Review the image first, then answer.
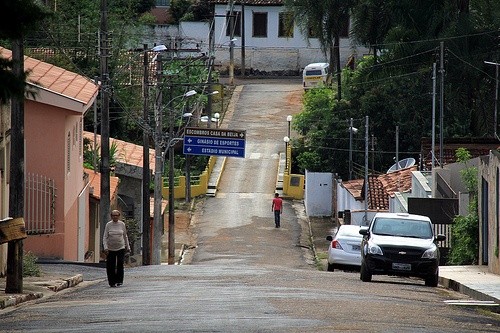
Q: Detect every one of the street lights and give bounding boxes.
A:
[99,44,168,256]
[141,89,221,267]
[283,136,290,169]
[286,114,293,140]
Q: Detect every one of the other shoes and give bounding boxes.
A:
[275,225,280,228]
[117,283,123,287]
[110,285,116,287]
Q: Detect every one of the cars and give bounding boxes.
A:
[325,224,369,272]
[359,212,447,288]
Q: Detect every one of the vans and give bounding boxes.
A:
[302,63,330,91]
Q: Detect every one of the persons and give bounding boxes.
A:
[103,210,131,287]
[272,193,283,228]
